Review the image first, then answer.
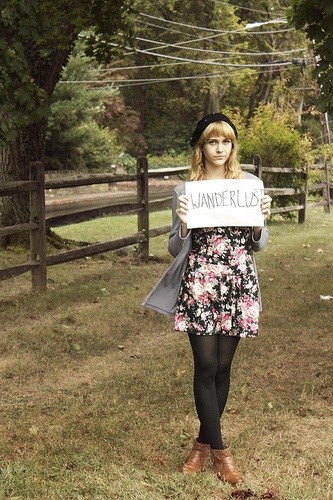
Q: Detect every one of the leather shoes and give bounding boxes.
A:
[181,437,244,486]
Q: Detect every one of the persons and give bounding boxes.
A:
[142,113,272,486]
